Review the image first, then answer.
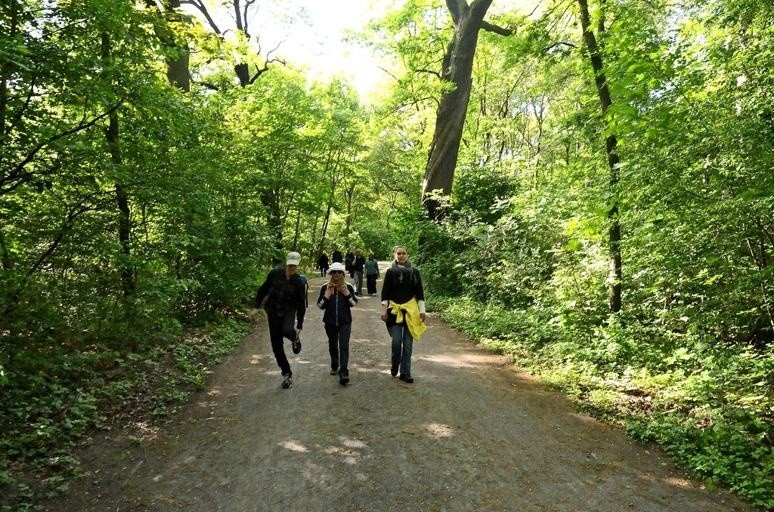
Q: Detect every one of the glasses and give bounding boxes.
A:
[331,271,343,274]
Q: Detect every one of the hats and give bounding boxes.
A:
[286,252,301,266]
[326,262,351,278]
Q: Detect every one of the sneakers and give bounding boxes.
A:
[399,374,413,383]
[292,335,301,354]
[391,365,398,377]
[330,368,338,375]
[368,293,376,297]
[340,376,349,384]
[281,372,292,388]
[355,292,362,297]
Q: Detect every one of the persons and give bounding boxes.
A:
[332,248,342,264]
[365,252,380,297]
[350,249,366,298]
[380,245,426,384]
[316,262,359,387]
[249,251,307,390]
[344,249,353,273]
[348,252,355,279]
[318,250,329,277]
